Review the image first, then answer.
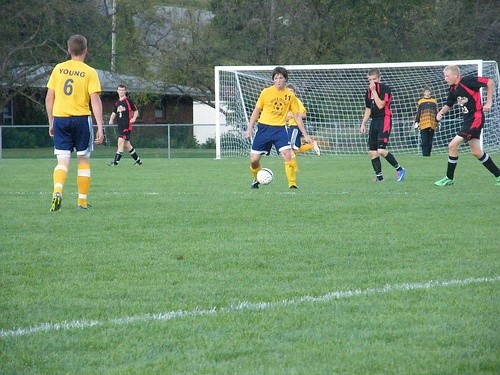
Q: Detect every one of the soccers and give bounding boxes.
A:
[256,167,275,186]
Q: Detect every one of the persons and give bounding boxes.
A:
[415,86,441,156]
[361,70,406,182]
[108,85,143,166]
[46,35,104,212]
[245,67,321,188]
[434,65,500,186]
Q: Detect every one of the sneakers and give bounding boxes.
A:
[49,191,62,212]
[76,203,92,211]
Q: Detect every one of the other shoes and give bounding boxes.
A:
[134,161,142,165]
[312,141,320,156]
[105,161,118,167]
[496,176,500,186]
[251,177,260,189]
[289,185,298,188]
[434,176,454,187]
[372,175,384,182]
[396,169,406,181]
